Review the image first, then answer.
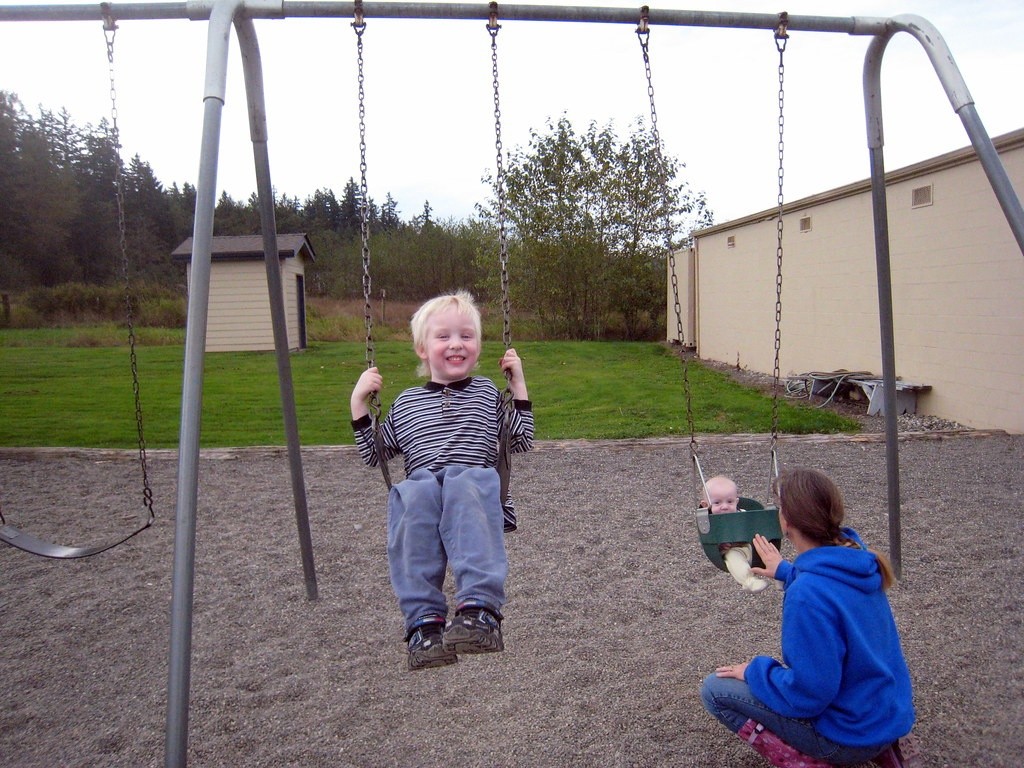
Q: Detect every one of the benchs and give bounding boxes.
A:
[805,374,932,417]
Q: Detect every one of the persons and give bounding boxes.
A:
[698,464,916,768]
[348,288,534,670]
[700,476,789,593]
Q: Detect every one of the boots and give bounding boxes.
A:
[872,731,921,768]
[739,719,831,768]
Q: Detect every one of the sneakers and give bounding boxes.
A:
[403,619,457,671]
[441,605,505,653]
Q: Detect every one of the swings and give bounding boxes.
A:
[0,23,158,560]
[639,32,784,574]
[355,26,522,534]
